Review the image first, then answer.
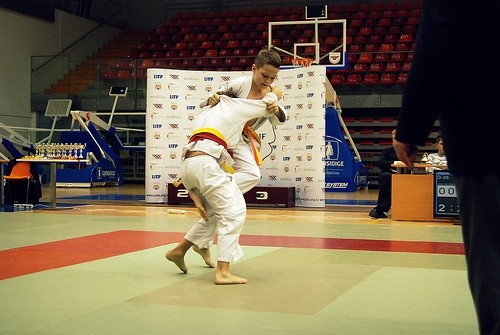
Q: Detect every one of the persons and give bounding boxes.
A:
[392,0,500,335]
[188,50,287,268]
[425,135,448,174]
[368,126,417,218]
[165,86,283,285]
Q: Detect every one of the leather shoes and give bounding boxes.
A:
[369,208,388,218]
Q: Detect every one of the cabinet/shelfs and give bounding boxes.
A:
[391,163,434,221]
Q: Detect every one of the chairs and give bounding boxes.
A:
[3,162,31,204]
[100,0,440,175]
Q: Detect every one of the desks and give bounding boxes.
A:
[16,159,89,210]
